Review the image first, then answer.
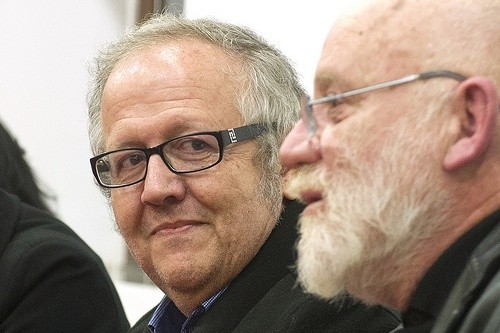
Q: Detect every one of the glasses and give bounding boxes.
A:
[305,70,468,140]
[90,123,277,188]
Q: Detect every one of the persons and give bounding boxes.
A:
[0,123,132,333]
[276,0,500,333]
[85,13,400,333]
